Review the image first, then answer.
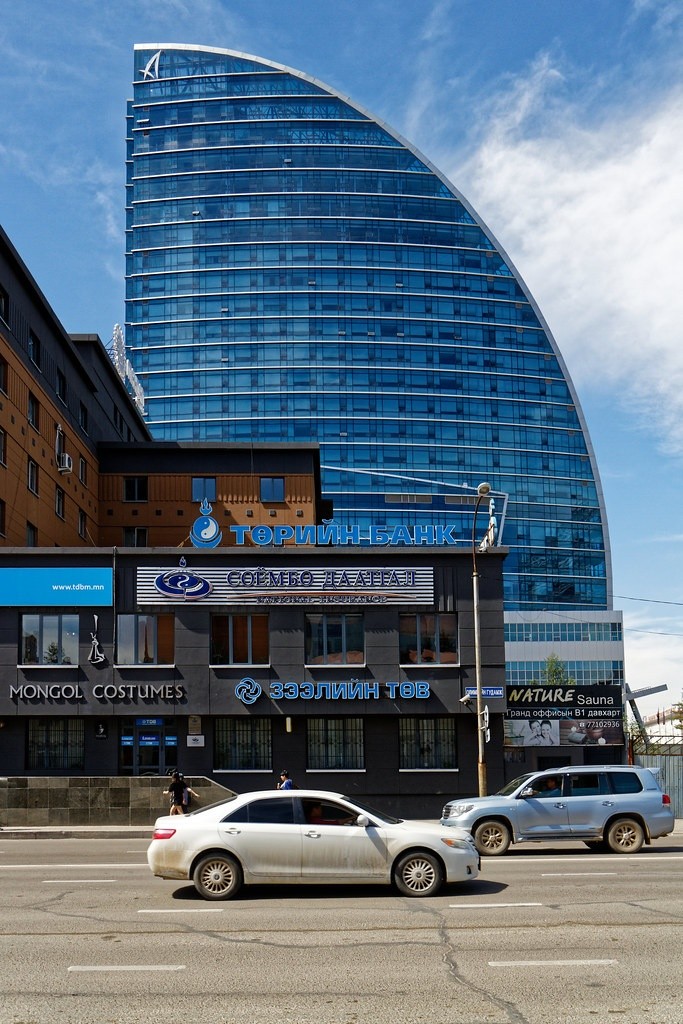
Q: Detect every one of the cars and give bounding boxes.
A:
[147,789,481,898]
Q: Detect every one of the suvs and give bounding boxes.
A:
[439,765,674,853]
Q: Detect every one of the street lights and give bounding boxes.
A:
[471,482,491,798]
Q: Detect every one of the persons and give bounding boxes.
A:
[521,720,543,744]
[163,771,200,815]
[177,772,189,814]
[526,720,558,745]
[302,799,350,827]
[276,769,293,789]
[530,775,562,799]
